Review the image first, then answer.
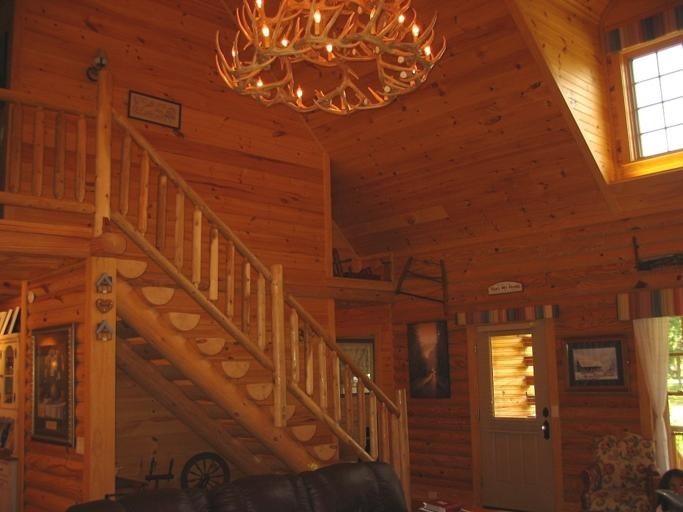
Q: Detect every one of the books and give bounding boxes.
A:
[422,498,463,512]
[0,306,20,336]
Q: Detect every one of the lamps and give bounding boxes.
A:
[214,0,447,115]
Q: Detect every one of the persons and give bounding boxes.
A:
[654,469,683,512]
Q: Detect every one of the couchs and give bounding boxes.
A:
[66,461,407,512]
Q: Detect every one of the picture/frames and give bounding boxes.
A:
[337,339,374,398]
[562,335,629,392]
[407,320,450,398]
[30,321,75,449]
[128,90,181,129]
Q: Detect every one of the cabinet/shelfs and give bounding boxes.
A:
[0,333,20,512]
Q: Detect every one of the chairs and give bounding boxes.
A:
[578,431,660,512]
